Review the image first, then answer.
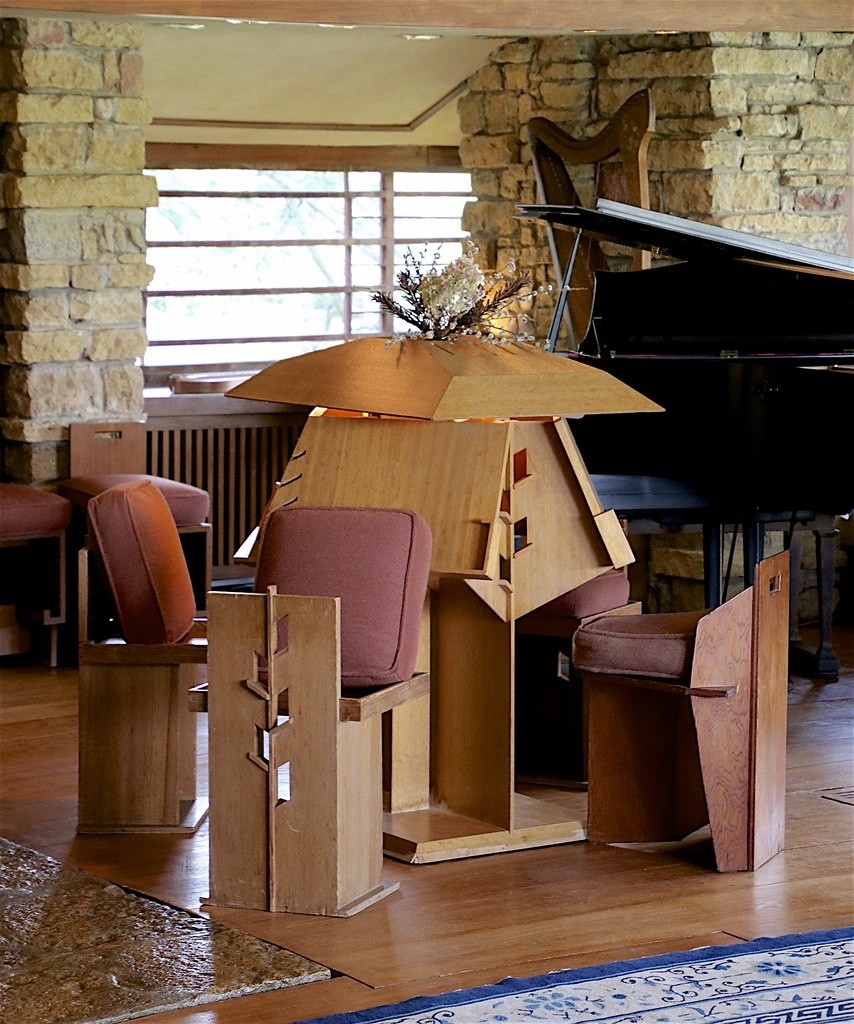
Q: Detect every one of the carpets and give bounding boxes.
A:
[295,926,854,1023]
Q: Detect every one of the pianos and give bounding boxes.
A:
[527,200,853,685]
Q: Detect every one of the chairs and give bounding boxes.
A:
[0,418,790,917]
